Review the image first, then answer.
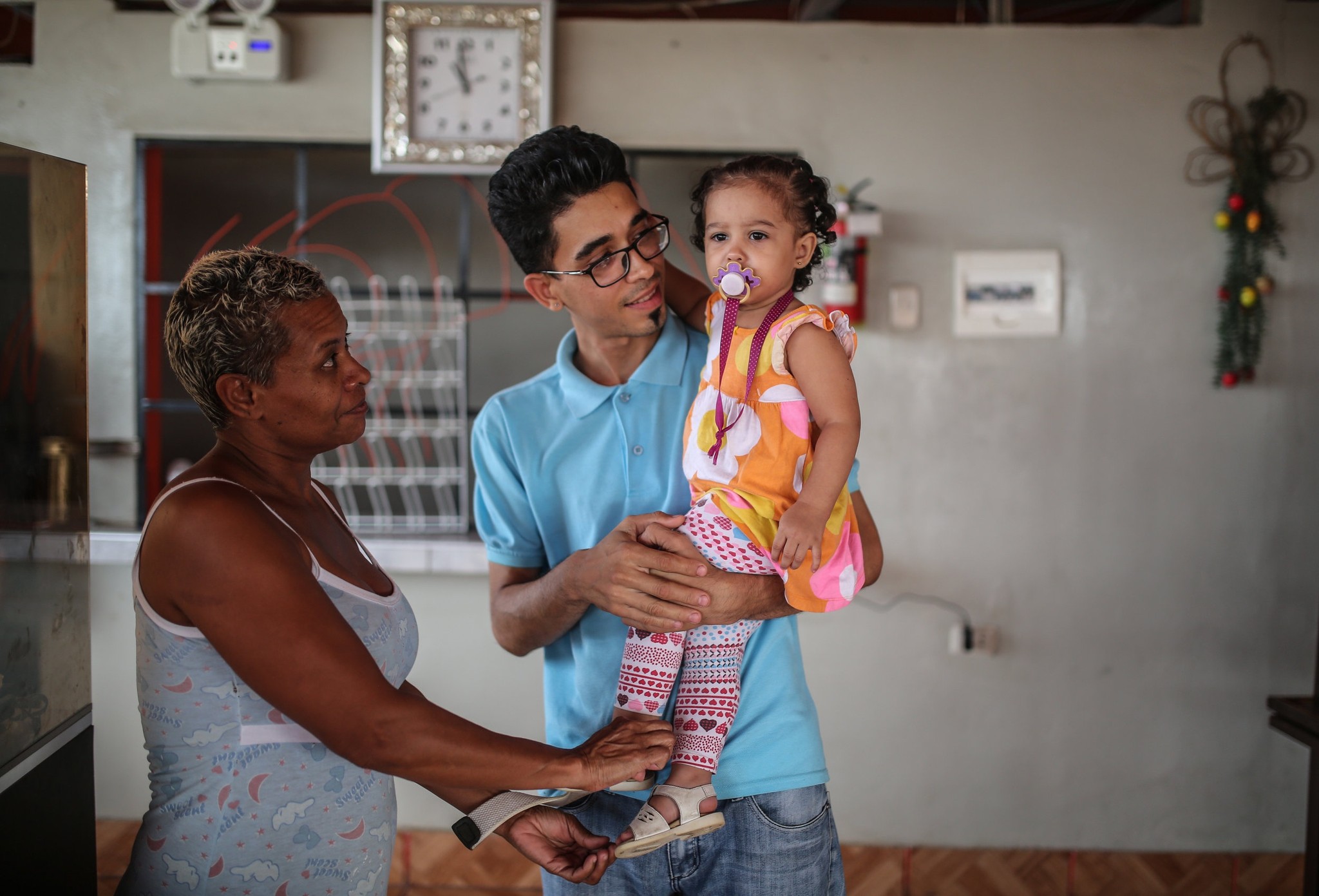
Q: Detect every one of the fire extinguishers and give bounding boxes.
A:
[817,176,877,322]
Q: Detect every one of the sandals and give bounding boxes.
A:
[614,784,725,858]
[554,772,655,792]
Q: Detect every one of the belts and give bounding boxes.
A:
[451,792,570,850]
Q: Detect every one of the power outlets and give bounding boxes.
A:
[950,626,1001,657]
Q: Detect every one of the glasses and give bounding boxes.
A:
[538,214,670,288]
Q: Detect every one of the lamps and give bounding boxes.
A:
[164,0,292,83]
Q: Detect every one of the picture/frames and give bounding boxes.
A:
[370,1,554,176]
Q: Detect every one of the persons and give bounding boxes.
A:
[558,156,861,858]
[471,123,883,896]
[117,247,675,896]
